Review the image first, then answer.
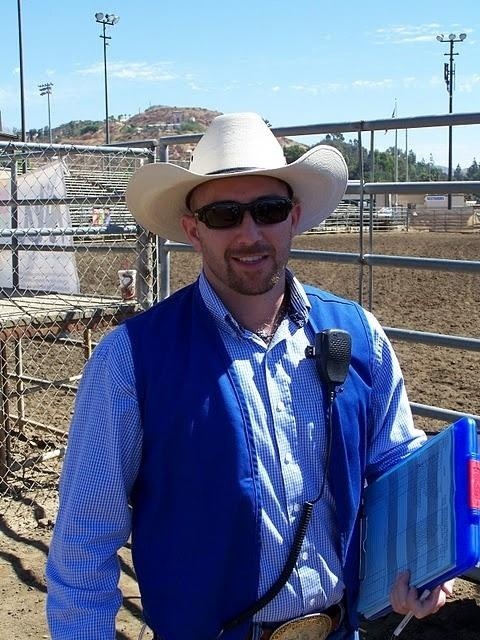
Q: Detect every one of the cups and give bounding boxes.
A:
[118,270,138,301]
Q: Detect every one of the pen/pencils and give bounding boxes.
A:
[390,589,432,639]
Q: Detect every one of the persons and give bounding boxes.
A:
[43,112,455,639]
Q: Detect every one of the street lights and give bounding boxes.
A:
[40,82,55,141]
[93,10,119,144]
[436,31,466,207]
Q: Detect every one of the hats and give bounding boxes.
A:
[125,112,349,246]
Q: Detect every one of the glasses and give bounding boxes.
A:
[197,194,292,229]
